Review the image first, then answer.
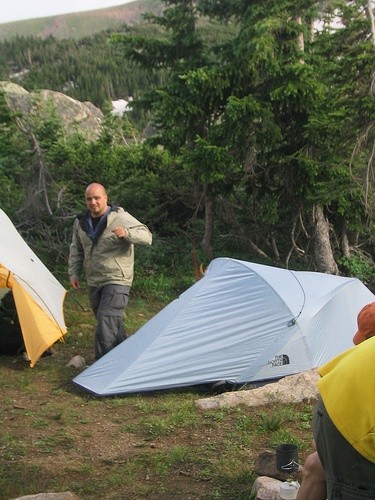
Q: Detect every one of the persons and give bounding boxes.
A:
[68,182,153,359]
[294,302,375,500]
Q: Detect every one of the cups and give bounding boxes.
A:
[276,444,298,471]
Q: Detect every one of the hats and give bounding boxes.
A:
[353,301,375,345]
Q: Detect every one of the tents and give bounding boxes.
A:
[0,208,87,368]
[72,257,375,395]
